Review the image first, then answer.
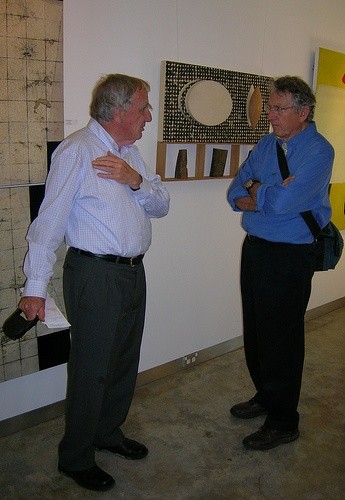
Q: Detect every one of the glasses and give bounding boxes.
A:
[264,105,295,113]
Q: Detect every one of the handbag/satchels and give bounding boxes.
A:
[312,221,343,271]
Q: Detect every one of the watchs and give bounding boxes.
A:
[245,179,259,192]
[132,176,143,191]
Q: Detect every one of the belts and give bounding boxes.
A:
[72,246,143,267]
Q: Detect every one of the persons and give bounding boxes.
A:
[226,75,334,449]
[20,74,170,492]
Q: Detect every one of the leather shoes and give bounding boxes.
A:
[229,393,266,418]
[60,465,115,490]
[95,435,148,460]
[242,423,300,450]
